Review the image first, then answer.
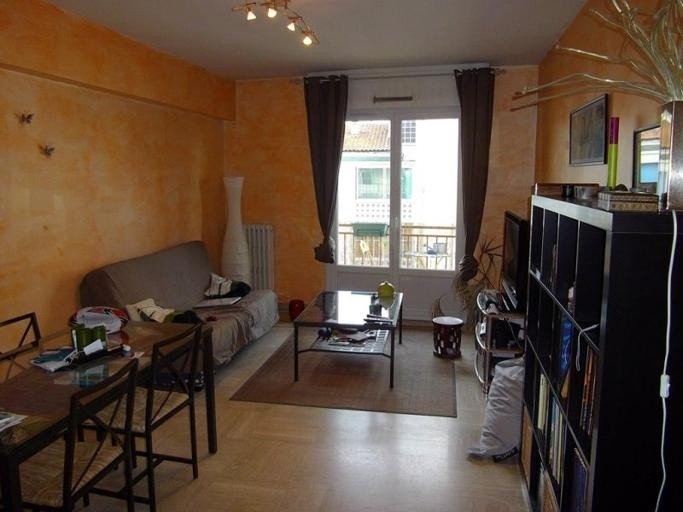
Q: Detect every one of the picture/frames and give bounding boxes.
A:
[568,92,608,168]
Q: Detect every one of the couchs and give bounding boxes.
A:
[79,240,280,393]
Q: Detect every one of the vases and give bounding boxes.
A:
[220,175,253,289]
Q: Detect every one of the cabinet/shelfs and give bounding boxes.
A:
[474,290,525,393]
[517,194,683,512]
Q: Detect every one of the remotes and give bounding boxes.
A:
[364,314,393,323]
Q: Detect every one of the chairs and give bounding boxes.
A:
[1,312,77,442]
[16,356,140,511]
[74,319,203,511]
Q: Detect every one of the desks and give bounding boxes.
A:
[2,318,214,505]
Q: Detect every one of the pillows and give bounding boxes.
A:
[125,298,174,323]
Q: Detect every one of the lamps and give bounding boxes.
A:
[231,0,320,47]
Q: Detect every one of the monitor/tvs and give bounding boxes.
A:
[499,211,528,312]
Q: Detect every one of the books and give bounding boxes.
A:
[538,345,598,485]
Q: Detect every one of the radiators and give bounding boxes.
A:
[242,223,276,291]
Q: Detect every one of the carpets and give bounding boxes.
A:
[229,324,458,419]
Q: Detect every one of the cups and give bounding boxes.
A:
[563,184,593,201]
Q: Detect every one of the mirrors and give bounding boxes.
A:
[631,122,661,193]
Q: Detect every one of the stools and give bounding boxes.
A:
[432,316,464,360]
